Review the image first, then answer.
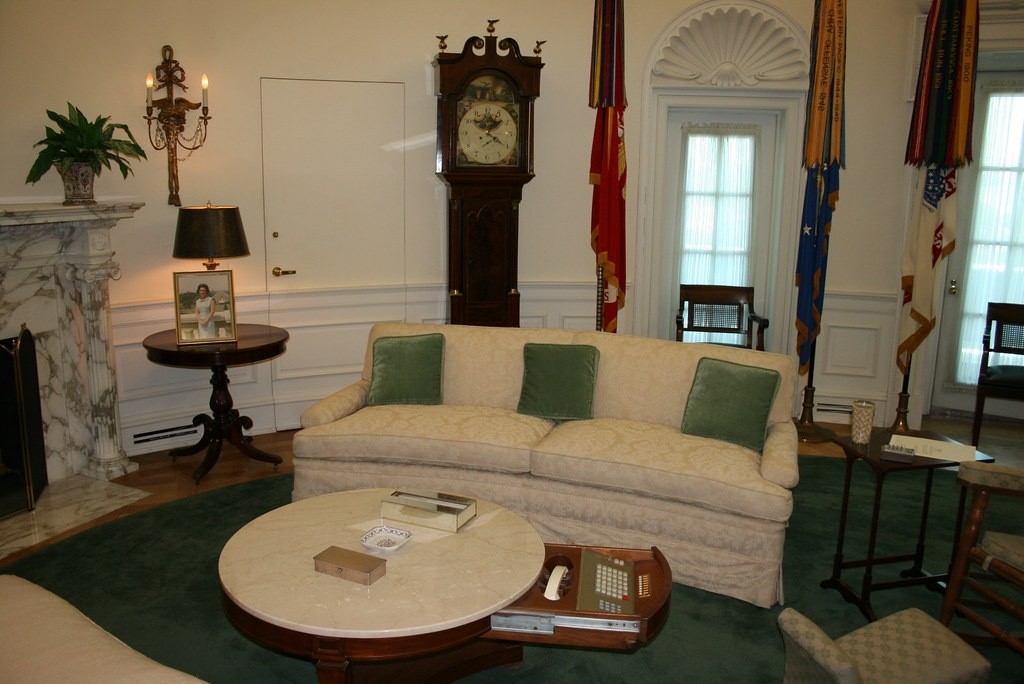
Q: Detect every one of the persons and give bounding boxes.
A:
[195,283,217,340]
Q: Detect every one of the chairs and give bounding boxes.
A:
[777,604,992,684]
[939,461,1024,661]
[972,302,1024,449]
[675,284,770,351]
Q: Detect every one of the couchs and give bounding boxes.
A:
[0,576,211,684]
[292,320,799,609]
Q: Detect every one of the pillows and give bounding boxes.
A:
[517,344,600,422]
[681,357,782,455]
[365,331,445,408]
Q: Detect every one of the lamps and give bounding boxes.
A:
[139,45,212,205]
[172,200,251,271]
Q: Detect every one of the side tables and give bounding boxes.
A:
[820,429,995,627]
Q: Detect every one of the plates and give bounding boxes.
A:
[360,525,412,553]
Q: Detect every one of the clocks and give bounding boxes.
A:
[429,19,547,328]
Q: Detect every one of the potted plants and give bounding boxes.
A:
[26,101,148,208]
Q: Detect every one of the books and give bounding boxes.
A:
[888,434,976,463]
[380,487,478,534]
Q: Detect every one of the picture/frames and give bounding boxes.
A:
[173,270,239,346]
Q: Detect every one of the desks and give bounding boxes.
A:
[143,323,289,486]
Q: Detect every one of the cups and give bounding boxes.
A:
[851,399,875,444]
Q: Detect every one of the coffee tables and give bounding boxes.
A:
[217,488,674,684]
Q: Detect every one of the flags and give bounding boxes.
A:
[793,0,847,377]
[894,0,981,373]
[587,0,629,336]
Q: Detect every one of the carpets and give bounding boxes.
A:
[0,452,1024,684]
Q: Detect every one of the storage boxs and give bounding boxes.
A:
[313,546,388,586]
